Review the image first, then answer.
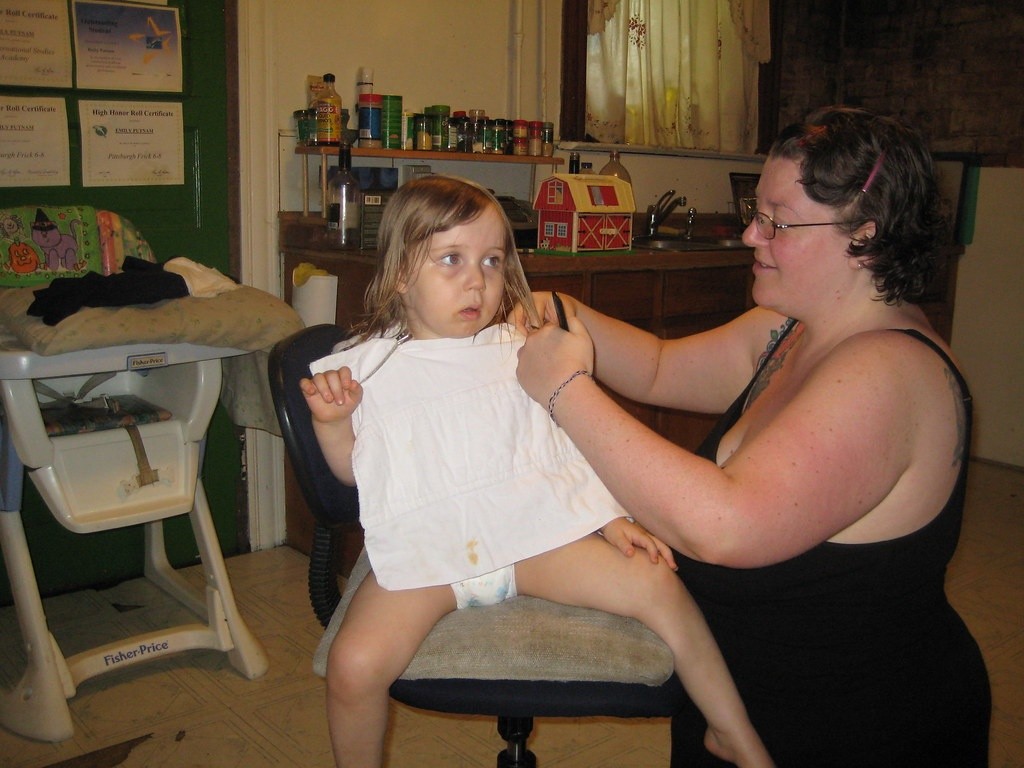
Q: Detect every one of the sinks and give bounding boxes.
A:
[634,239,726,251]
[693,238,748,248]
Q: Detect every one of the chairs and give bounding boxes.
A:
[266,321,692,768]
[1,205,267,746]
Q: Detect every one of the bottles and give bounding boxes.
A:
[293,67,554,157]
[568,151,580,176]
[579,162,596,175]
[599,152,632,189]
[325,141,361,249]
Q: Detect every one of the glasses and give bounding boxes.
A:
[738,195,870,240]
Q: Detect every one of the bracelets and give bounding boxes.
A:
[547,370,591,429]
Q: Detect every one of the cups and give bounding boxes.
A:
[739,198,757,226]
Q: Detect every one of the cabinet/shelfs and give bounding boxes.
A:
[279,146,758,453]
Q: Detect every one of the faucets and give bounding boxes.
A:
[645,189,687,237]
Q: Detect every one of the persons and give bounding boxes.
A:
[298,171,780,768]
[511,104,994,768]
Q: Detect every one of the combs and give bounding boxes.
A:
[551,290,570,332]
